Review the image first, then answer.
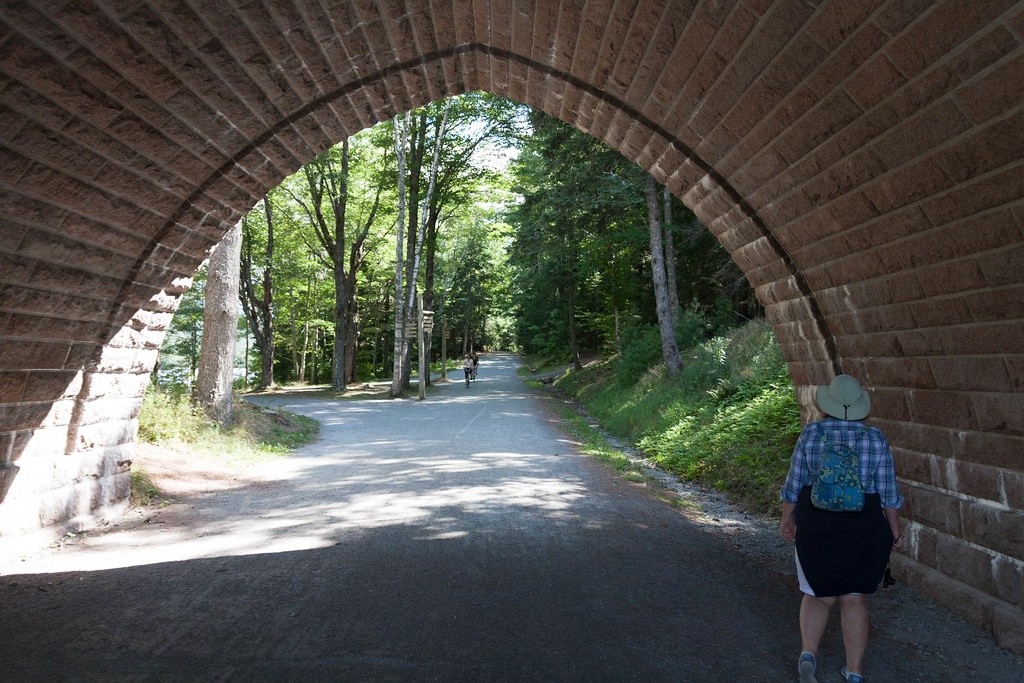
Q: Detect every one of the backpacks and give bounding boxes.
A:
[807,421,876,510]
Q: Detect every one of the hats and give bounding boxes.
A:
[816,373,871,420]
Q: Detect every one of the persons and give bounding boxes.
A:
[463,352,478,385]
[781,374,905,683]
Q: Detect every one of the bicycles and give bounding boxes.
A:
[463,367,471,388]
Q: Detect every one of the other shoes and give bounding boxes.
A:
[799,652,817,683]
[841,666,862,683]
